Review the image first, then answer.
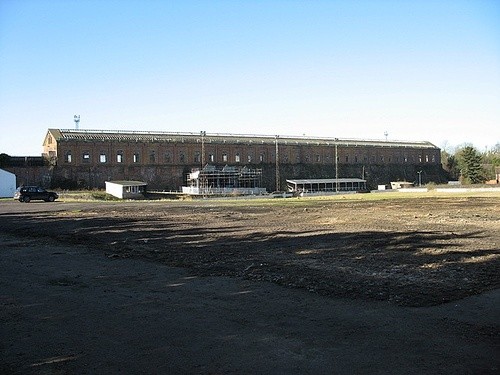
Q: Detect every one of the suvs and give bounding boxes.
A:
[14,186,59,203]
